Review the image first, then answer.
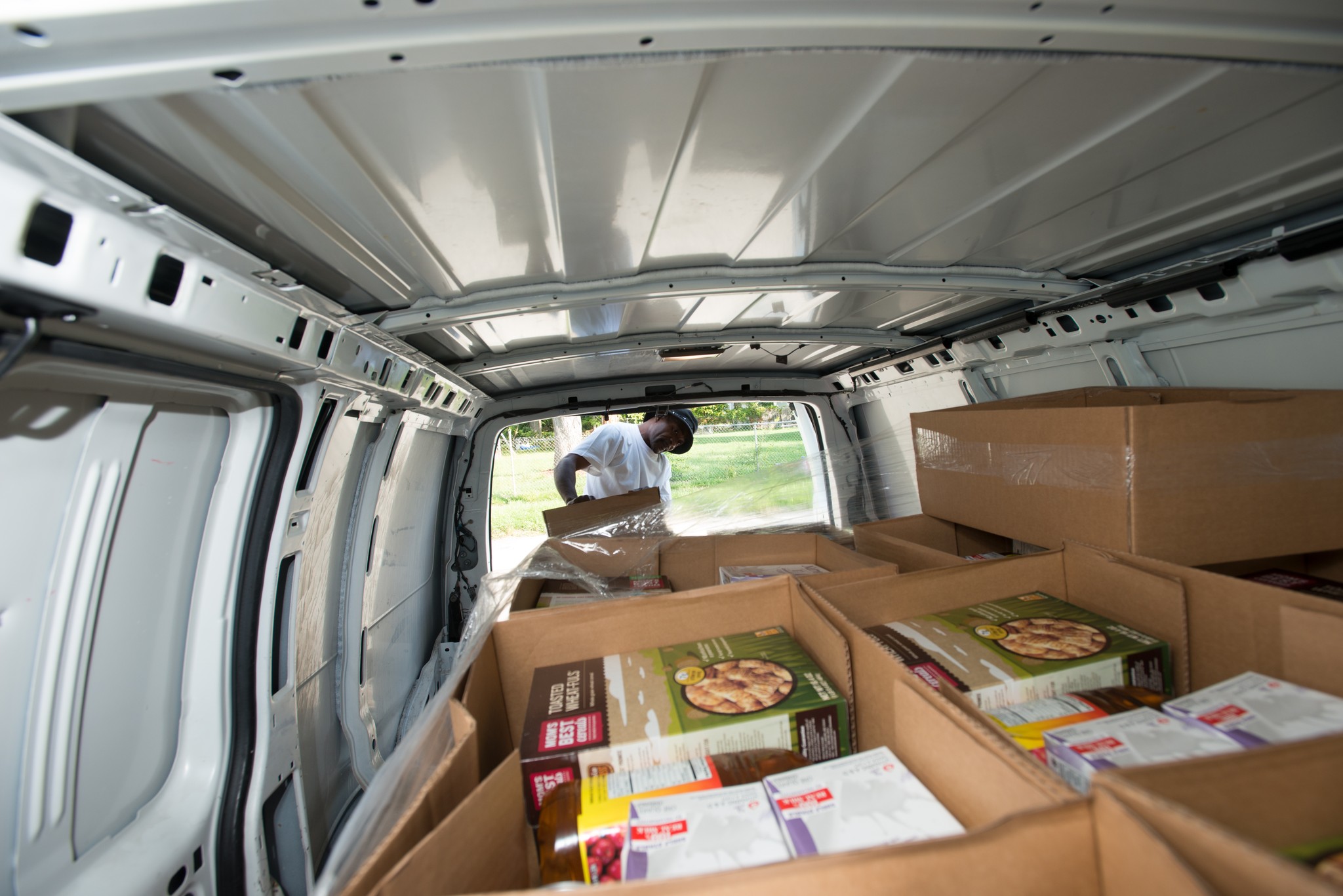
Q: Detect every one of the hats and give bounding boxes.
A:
[642,408,698,455]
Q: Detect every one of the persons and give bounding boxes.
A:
[553,407,697,509]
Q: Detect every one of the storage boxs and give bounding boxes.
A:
[340,385,1341,894]
